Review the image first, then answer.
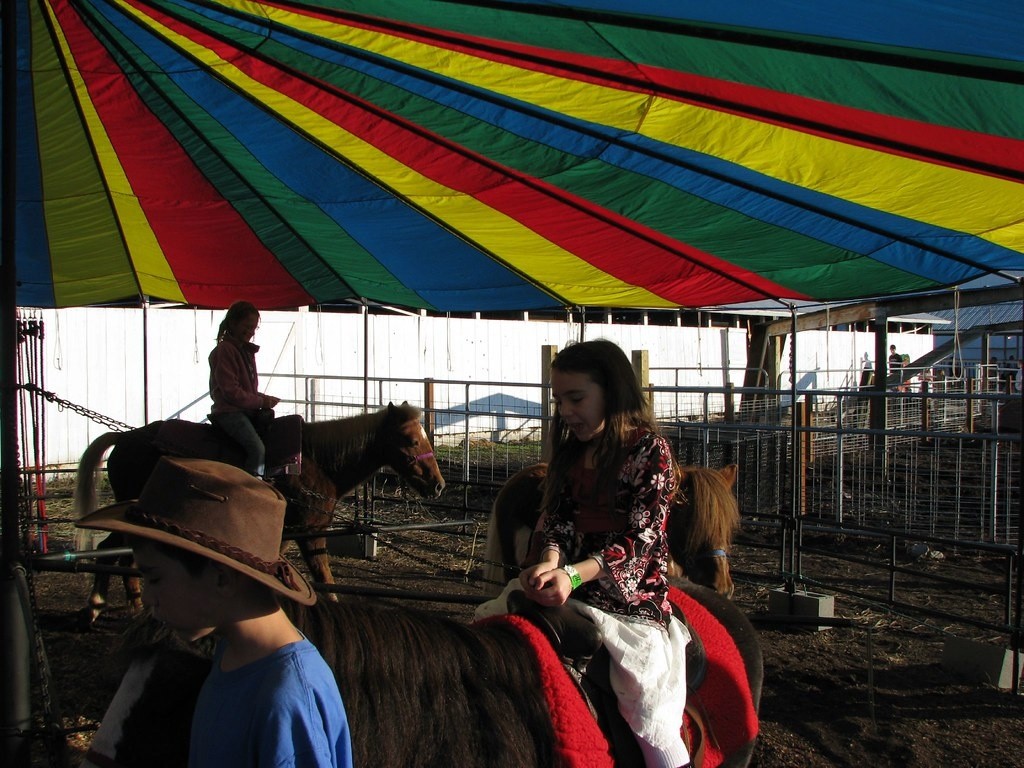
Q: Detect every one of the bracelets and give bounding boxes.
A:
[563,565,583,590]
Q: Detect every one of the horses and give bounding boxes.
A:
[485,463,739,599]
[73,402,446,629]
[77,587,763,768]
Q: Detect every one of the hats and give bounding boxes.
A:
[74,455,317,605]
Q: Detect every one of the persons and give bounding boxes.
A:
[74,453,353,768]
[471,340,693,767]
[948,354,1023,394]
[889,345,903,373]
[208,302,279,477]
